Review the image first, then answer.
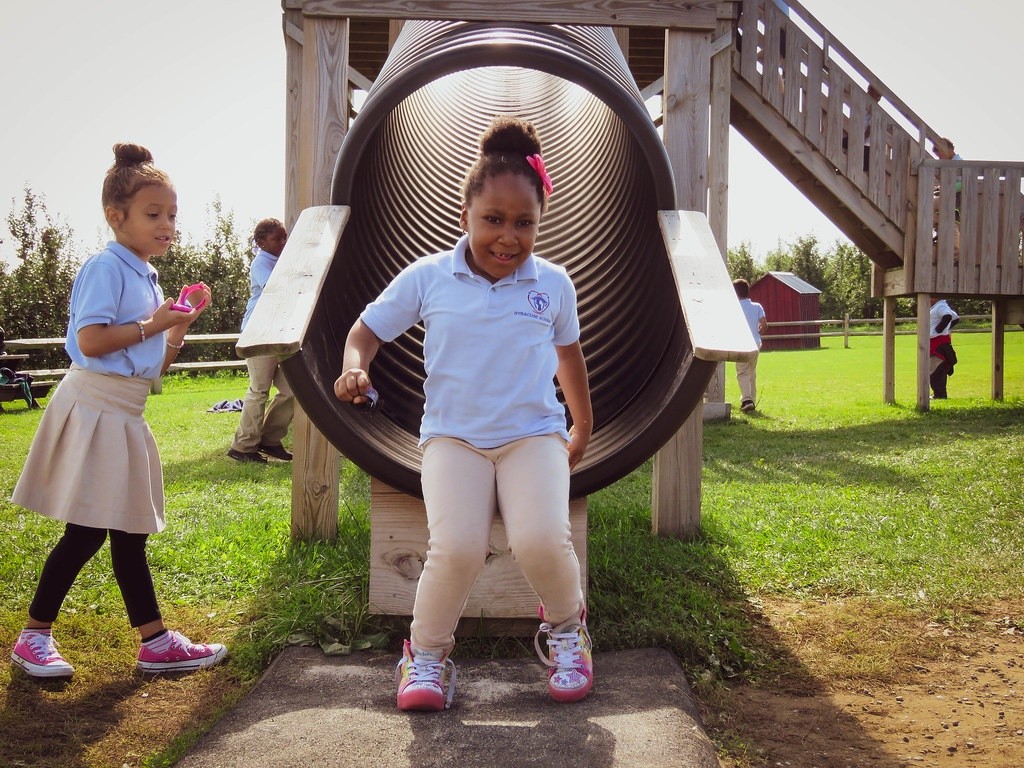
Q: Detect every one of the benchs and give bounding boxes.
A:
[0,381,58,414]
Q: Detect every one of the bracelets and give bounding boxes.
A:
[137,321,145,343]
[167,340,185,349]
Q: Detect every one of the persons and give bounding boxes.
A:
[226,220,295,463]
[732,278,769,412]
[334,115,593,709]
[928,294,959,400]
[10,140,227,678]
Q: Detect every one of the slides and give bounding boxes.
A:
[280,18,719,501]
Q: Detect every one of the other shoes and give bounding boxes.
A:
[740,400,755,411]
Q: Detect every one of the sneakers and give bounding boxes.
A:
[136,632,228,673]
[534,601,593,701]
[257,444,293,460]
[395,639,456,710]
[10,633,76,677]
[226,448,267,464]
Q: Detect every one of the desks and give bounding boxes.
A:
[0,354,29,380]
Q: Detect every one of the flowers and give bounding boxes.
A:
[526,154,553,194]
